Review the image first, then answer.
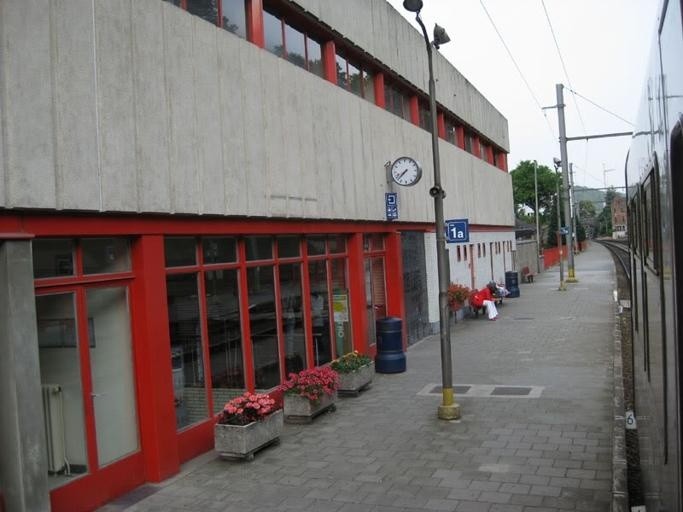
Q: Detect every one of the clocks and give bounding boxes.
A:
[389,155,423,187]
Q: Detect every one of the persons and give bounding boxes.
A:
[470,289,499,321]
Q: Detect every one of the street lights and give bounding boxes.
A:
[550,155,570,290]
[399,0,466,424]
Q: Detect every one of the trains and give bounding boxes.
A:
[620,0,682,512]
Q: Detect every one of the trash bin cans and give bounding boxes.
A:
[505,271,520,297]
[374,316,406,373]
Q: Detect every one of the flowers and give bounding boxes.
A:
[267,365,340,407]
[214,388,278,427]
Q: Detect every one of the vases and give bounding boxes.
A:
[273,381,338,425]
[204,406,285,464]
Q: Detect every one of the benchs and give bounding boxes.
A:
[466,282,506,317]
[521,265,537,282]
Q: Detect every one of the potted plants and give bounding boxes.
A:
[328,348,377,403]
